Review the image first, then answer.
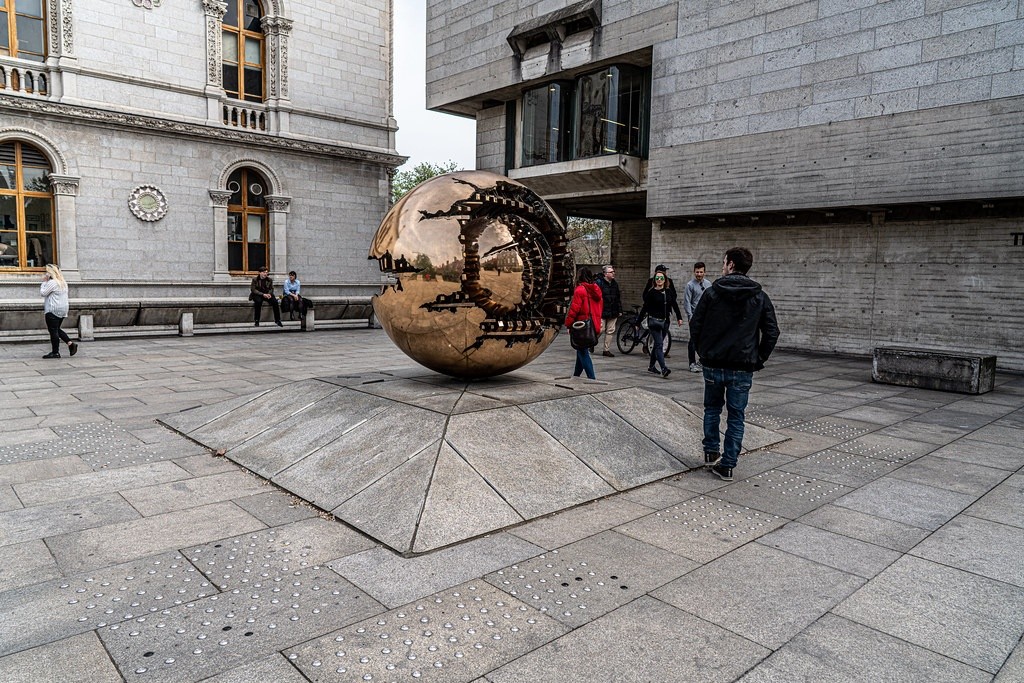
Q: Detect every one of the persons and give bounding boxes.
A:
[589,264,623,357]
[426,273,430,280]
[634,270,684,378]
[283,271,304,320]
[248,267,283,327]
[642,264,677,358]
[684,262,713,373]
[498,268,500,276]
[564,266,603,380]
[0,243,18,267]
[40,263,78,359]
[689,246,780,480]
[27,235,50,267]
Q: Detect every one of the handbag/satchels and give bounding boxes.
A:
[648,317,666,330]
[570,318,598,351]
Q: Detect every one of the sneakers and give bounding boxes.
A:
[712,465,733,480]
[704,452,720,464]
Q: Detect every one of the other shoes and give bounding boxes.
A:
[665,354,670,358]
[643,346,648,354]
[589,346,594,353]
[43,352,60,358]
[256,321,259,326]
[602,351,614,357]
[648,365,661,374]
[275,321,283,327]
[689,363,699,373]
[662,366,671,377]
[696,362,703,372]
[69,343,77,356]
[290,315,295,320]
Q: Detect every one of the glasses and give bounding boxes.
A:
[606,270,615,273]
[699,282,705,291]
[655,276,664,281]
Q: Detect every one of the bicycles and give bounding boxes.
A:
[617,304,672,358]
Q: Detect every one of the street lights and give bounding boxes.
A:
[412,252,419,266]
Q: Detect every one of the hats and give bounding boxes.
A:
[655,265,669,271]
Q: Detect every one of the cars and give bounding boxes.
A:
[484,263,494,271]
